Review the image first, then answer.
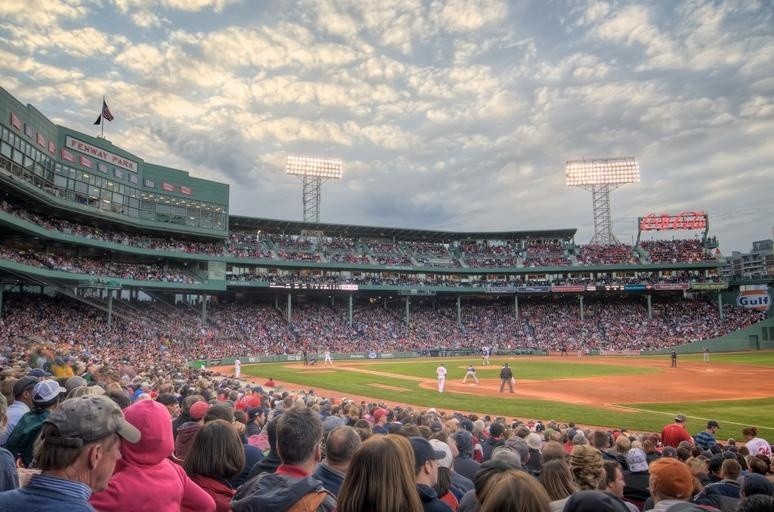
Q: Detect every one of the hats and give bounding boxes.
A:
[245,407,262,424]
[570,444,603,465]
[66,376,87,390]
[728,437,735,443]
[454,429,473,449]
[182,394,204,407]
[568,429,576,439]
[28,368,51,377]
[707,420,720,429]
[571,430,585,443]
[428,438,452,468]
[408,436,445,466]
[189,401,212,418]
[157,393,175,405]
[66,386,95,398]
[678,440,690,449]
[648,457,692,497]
[218,389,223,394]
[543,428,555,439]
[101,370,112,376]
[649,433,660,444]
[662,446,676,457]
[626,447,648,471]
[709,455,721,470]
[373,407,388,416]
[141,381,150,387]
[31,379,65,402]
[13,375,38,395]
[526,432,542,455]
[41,394,141,448]
[102,381,129,398]
[322,415,345,433]
[562,488,629,512]
[432,422,441,431]
[723,458,739,473]
[254,385,266,394]
[674,414,686,421]
[492,446,521,465]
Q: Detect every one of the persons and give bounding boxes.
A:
[480,469,549,512]
[459,446,520,512]
[0,379,38,447]
[0,392,20,491]
[474,460,509,504]
[90,399,216,512]
[541,461,574,502]
[4,378,66,467]
[234,357,241,377]
[452,430,481,482]
[593,414,738,458]
[463,364,479,384]
[499,362,515,393]
[694,459,742,512]
[406,437,453,512]
[549,444,605,512]
[175,401,212,460]
[232,407,338,512]
[246,406,266,437]
[505,436,540,476]
[738,453,774,512]
[182,419,246,512]
[601,460,626,498]
[457,412,593,448]
[623,448,650,502]
[1,184,768,364]
[322,393,457,436]
[428,438,459,512]
[336,434,424,512]
[311,426,361,497]
[644,458,693,512]
[0,352,235,377]
[644,458,722,512]
[741,427,772,458]
[68,377,322,411]
[436,363,447,392]
[0,394,141,512]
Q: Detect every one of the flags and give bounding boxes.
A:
[104,100,114,122]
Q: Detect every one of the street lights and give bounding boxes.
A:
[286,155,343,222]
[566,161,640,244]
